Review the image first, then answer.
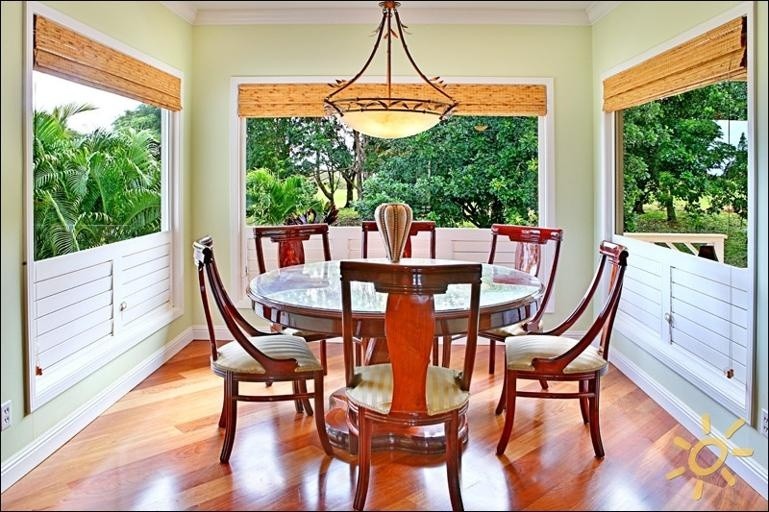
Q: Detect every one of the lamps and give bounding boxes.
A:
[324,1,458,139]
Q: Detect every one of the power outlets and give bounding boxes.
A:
[1,400,12,430]
[760,408,769,438]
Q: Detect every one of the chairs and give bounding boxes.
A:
[356,221,438,366]
[192,235,334,463]
[253,224,361,386]
[496,241,629,457]
[325,260,482,511]
[442,224,563,389]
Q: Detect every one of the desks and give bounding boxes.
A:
[246,258,546,454]
